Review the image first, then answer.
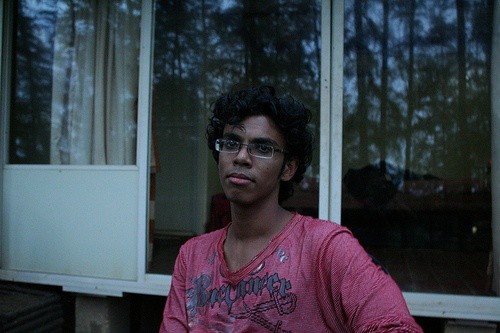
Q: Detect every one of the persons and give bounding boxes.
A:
[157,71,428,333]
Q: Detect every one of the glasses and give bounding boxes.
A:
[215,138,284,157]
